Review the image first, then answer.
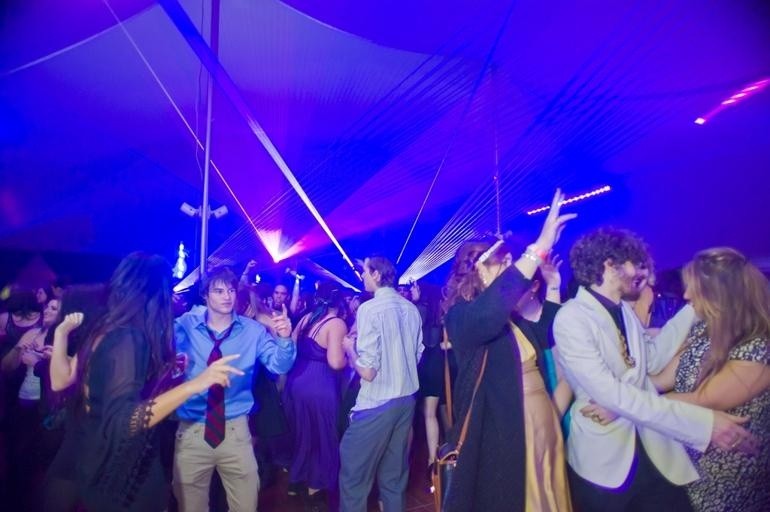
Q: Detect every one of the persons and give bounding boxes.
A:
[443,188,576,511]
[550,228,770,511]
[238,255,445,511]
[0,251,296,512]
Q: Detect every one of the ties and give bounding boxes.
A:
[202,326,234,449]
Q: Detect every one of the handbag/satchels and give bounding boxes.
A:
[429,442,470,508]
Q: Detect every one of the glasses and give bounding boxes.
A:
[211,288,239,296]
